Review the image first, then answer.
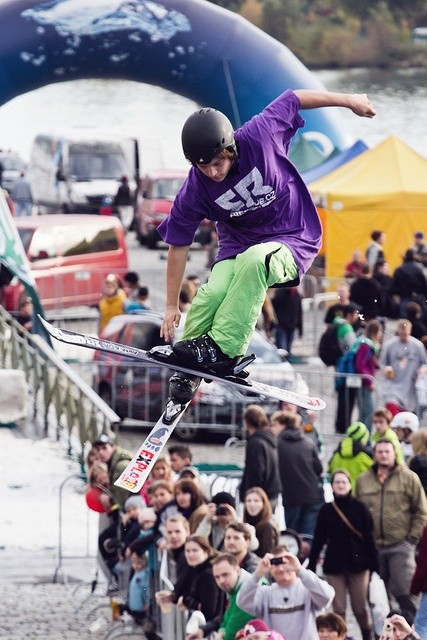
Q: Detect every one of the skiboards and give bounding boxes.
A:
[34,312,325,496]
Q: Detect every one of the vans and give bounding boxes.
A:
[0,148,28,194]
[0,213,131,320]
[91,309,315,441]
[136,172,213,249]
[28,132,137,218]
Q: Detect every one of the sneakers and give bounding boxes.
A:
[173,334,244,374]
[169,371,202,404]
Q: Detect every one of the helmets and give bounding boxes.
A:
[182,108,236,168]
[390,412,420,433]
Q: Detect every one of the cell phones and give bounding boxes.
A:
[270,557,283,565]
[216,507,227,516]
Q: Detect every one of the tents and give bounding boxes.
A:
[306,132,427,288]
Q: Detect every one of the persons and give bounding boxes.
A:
[177,535,228,620]
[356,438,427,622]
[195,502,238,552]
[307,470,378,640]
[168,278,193,348]
[178,466,200,487]
[407,429,427,493]
[97,275,125,339]
[222,522,262,574]
[185,553,271,640]
[154,515,191,603]
[151,89,376,376]
[88,461,111,494]
[129,551,155,639]
[316,612,353,640]
[115,176,134,234]
[123,271,141,300]
[137,507,158,578]
[405,302,427,345]
[329,422,374,500]
[412,231,426,266]
[11,172,34,217]
[245,618,286,640]
[394,249,427,319]
[351,265,384,367]
[377,610,420,640]
[277,397,322,455]
[124,286,151,313]
[175,479,211,534]
[372,257,403,365]
[270,284,303,357]
[13,296,35,394]
[87,451,99,468]
[187,274,201,294]
[243,487,280,557]
[370,406,406,467]
[168,445,200,483]
[236,545,335,640]
[146,484,162,513]
[375,318,427,429]
[393,412,418,470]
[138,457,176,508]
[365,230,386,267]
[235,629,251,640]
[117,495,149,581]
[334,303,362,435]
[322,283,363,332]
[343,250,372,284]
[154,480,177,561]
[237,405,280,524]
[358,319,384,433]
[93,434,133,595]
[206,218,220,268]
[270,411,326,554]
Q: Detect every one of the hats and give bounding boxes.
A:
[138,507,157,525]
[93,434,114,445]
[244,619,271,638]
[180,466,199,477]
[125,495,147,509]
[347,422,369,446]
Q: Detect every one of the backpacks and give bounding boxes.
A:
[335,343,371,395]
[319,324,344,364]
